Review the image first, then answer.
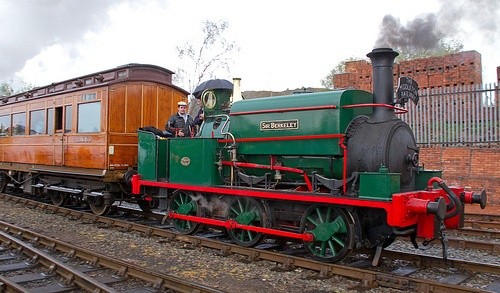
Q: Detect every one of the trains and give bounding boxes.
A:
[0,48,487,271]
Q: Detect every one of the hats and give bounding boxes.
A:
[177,101,187,105]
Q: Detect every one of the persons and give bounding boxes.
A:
[165,101,194,138]
[191,98,203,127]
[35,110,45,135]
